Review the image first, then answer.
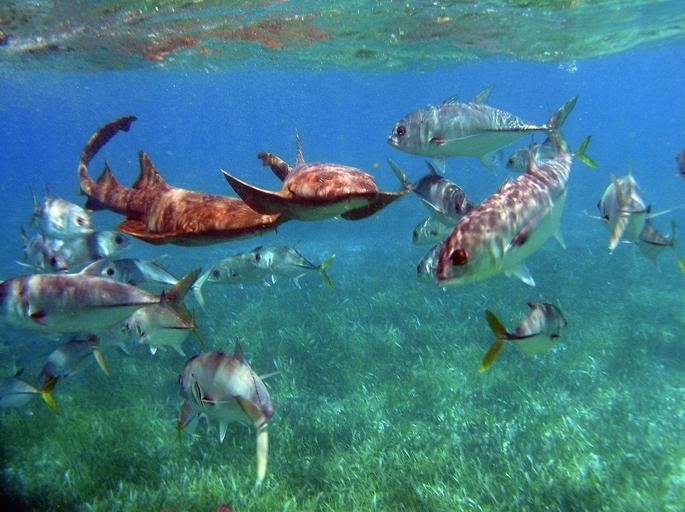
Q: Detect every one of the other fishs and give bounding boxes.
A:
[385,83,598,294]
[0,180,337,491]
